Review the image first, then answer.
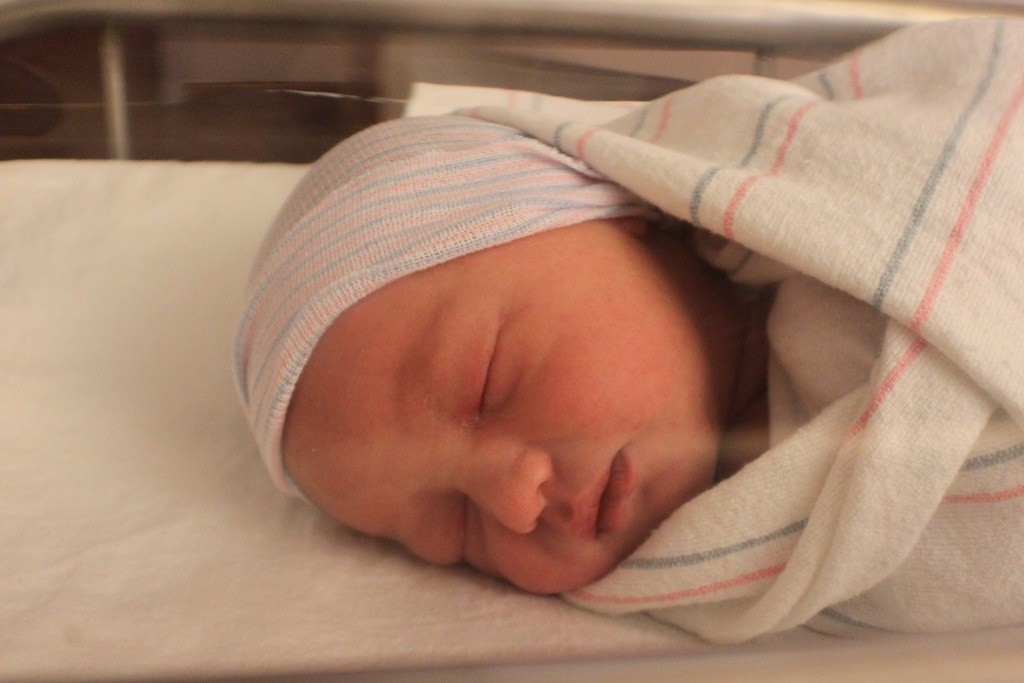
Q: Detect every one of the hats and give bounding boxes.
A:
[234,80,664,517]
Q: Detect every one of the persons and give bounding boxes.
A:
[230,15,1024,647]
